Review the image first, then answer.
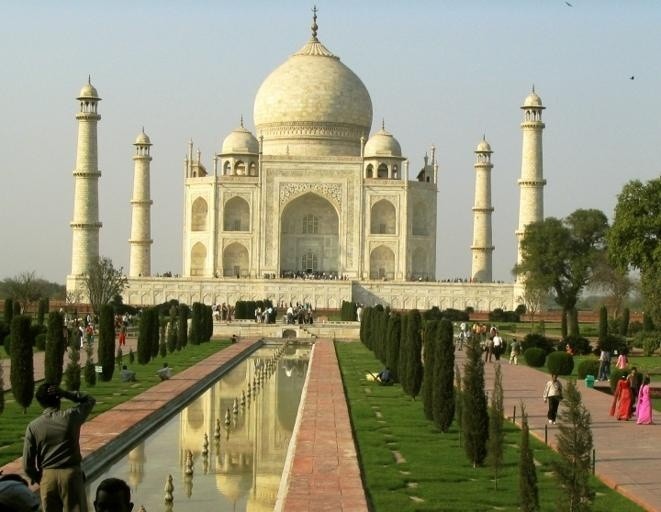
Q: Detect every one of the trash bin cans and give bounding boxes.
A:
[585,374,595,387]
[87,335,95,342]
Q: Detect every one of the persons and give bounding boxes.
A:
[608,372,633,422]
[615,350,628,370]
[138,270,516,284]
[373,366,396,386]
[119,364,136,384]
[155,362,170,380]
[542,372,563,426]
[92,478,134,511]
[624,367,639,414]
[563,342,575,356]
[633,374,654,425]
[211,300,314,324]
[229,333,238,344]
[603,347,611,382]
[452,319,520,366]
[356,303,366,322]
[19,382,95,512]
[0,472,40,511]
[62,304,149,352]
[593,348,607,382]
